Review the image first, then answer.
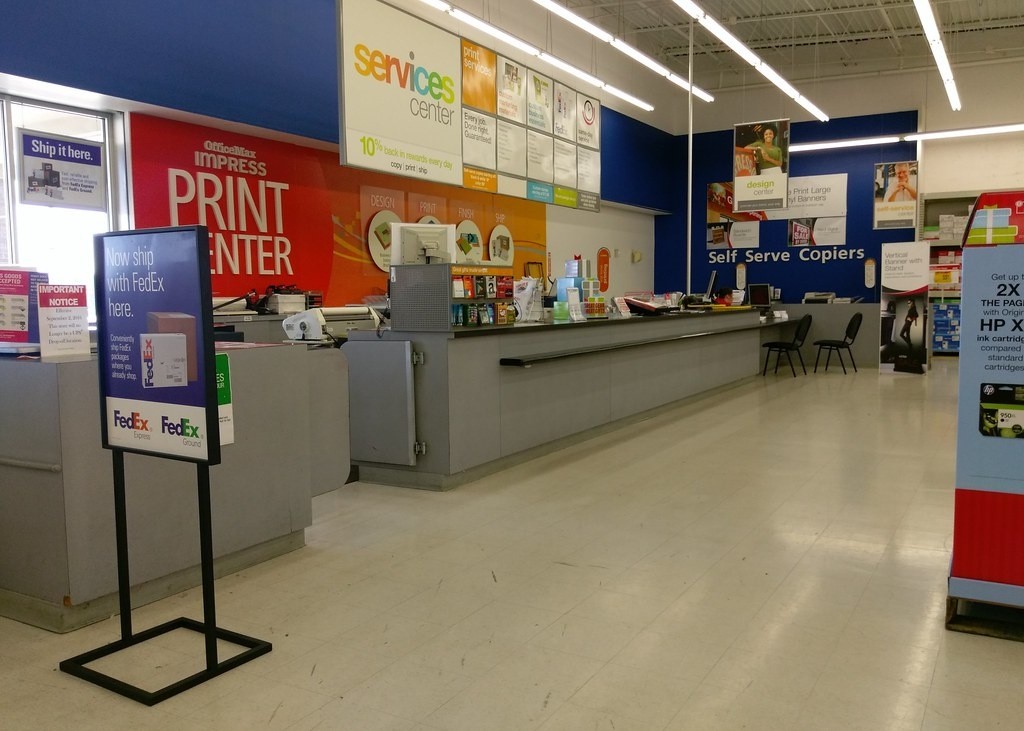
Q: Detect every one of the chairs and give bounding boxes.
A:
[761,313,812,377]
[812,312,863,375]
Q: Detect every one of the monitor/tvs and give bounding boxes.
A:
[392,222,456,265]
[706,271,719,299]
[748,283,771,308]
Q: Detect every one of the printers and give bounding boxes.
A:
[802,292,836,304]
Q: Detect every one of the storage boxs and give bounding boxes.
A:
[954,216,969,228]
[955,250,962,264]
[939,227,954,240]
[934,319,960,334]
[953,227,966,241]
[933,333,960,351]
[933,304,961,319]
[930,250,955,264]
[924,226,939,239]
[938,214,955,228]
[929,265,960,284]
[259,294,305,314]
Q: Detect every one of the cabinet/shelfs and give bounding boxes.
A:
[918,186,1024,353]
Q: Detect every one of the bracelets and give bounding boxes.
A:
[757,146,760,151]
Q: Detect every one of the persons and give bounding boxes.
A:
[899,299,919,350]
[745,126,785,175]
[877,163,917,202]
